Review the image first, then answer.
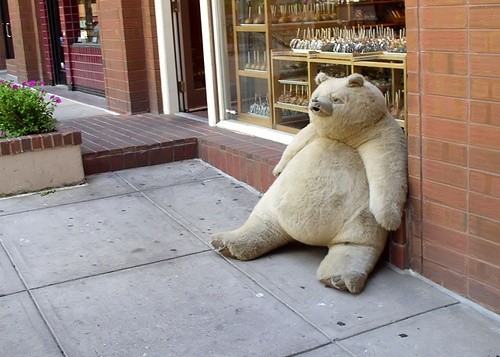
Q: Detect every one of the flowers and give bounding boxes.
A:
[0,77,62,137]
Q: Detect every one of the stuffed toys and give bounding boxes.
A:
[211,72,407,294]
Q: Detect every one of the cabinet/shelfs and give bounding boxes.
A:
[232,0,406,131]
[79,16,101,45]
[271,45,408,148]
